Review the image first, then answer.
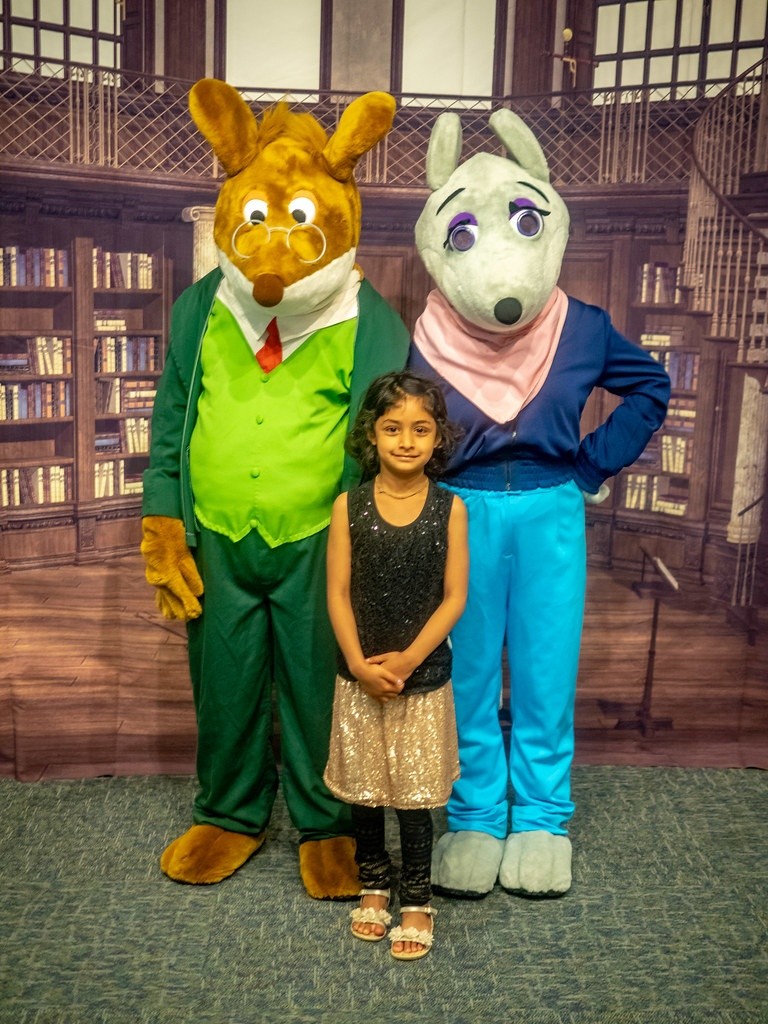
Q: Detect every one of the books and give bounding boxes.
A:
[1,466,72,506]
[638,259,685,304]
[663,398,697,430]
[625,473,688,517]
[640,323,684,347]
[94,459,143,498]
[94,308,126,332]
[95,377,157,412]
[649,350,700,392]
[637,435,694,476]
[95,417,148,454]
[0,336,71,375]
[93,241,154,289]
[93,336,158,372]
[0,244,70,287]
[0,380,72,420]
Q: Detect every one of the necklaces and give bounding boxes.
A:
[374,473,429,499]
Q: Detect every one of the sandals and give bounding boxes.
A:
[349,888,392,942]
[387,906,438,960]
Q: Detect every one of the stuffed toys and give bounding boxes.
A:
[403,107,672,900]
[139,76,411,901]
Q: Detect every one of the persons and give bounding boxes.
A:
[325,370,470,960]
